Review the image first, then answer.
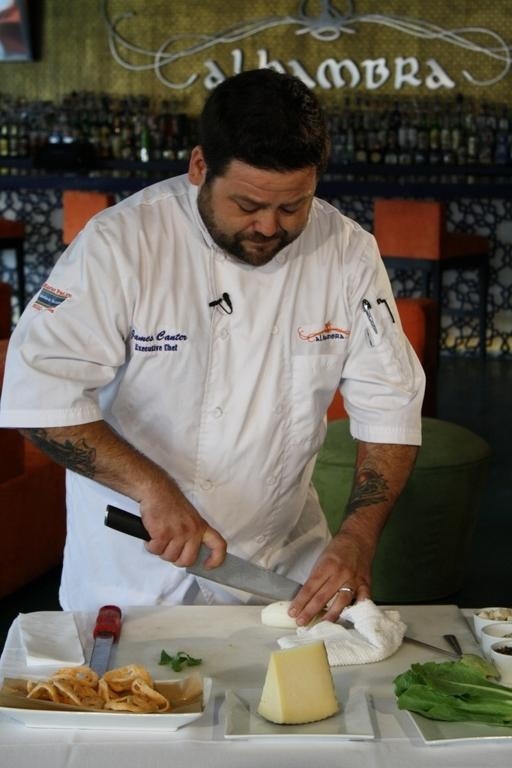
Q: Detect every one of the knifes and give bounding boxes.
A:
[102,504,304,603]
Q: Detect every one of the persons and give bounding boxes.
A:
[0,71,429,627]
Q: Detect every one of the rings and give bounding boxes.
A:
[338,586,355,593]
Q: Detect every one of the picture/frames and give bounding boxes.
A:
[0,1,35,64]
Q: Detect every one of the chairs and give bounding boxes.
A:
[0,189,115,320]
[329,193,494,420]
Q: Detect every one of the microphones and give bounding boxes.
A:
[222,293,232,307]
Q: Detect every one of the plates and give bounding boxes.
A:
[1,674,216,733]
[399,709,512,746]
[223,687,375,740]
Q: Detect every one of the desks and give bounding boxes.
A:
[0,602,511,768]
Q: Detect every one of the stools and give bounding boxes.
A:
[313,414,490,603]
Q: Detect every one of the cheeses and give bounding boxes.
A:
[257,640,340,725]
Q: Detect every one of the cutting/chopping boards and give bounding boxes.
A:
[111,605,496,699]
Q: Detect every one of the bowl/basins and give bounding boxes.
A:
[472,607,512,689]
